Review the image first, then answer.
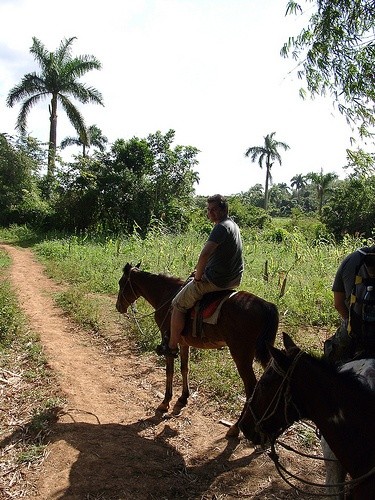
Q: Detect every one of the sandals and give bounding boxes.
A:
[157,343,179,358]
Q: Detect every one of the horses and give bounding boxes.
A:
[236,333,375,500]
[115,261,280,415]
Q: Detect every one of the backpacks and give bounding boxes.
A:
[345,249,375,343]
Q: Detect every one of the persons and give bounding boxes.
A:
[155,193,243,356]
[332,244,374,361]
